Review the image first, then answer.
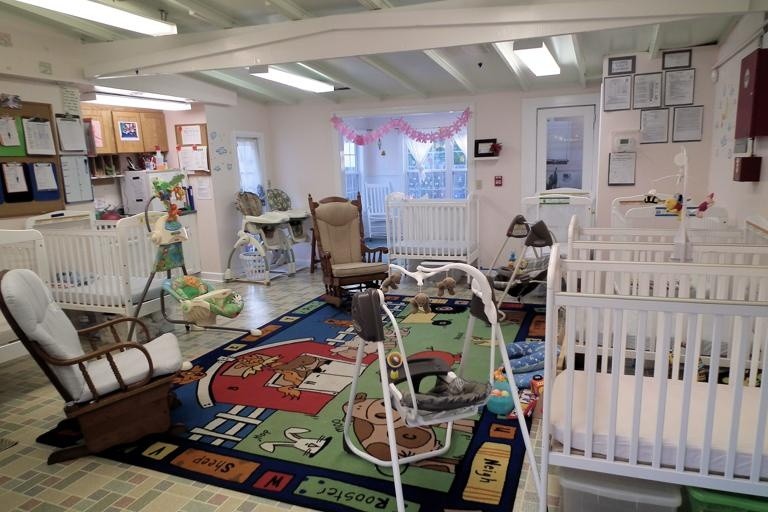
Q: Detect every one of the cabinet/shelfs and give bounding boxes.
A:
[80,102,169,157]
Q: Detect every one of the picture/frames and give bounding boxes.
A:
[662,49,692,70]
[609,56,636,76]
[474,140,500,157]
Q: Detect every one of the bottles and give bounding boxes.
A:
[155,149,163,169]
[163,159,168,169]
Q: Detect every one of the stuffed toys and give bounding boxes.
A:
[643,189,659,204]
[437,277,456,297]
[696,192,716,219]
[411,293,432,314]
[382,270,402,293]
[485,341,562,419]
[664,198,682,221]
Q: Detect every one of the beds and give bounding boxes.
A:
[0,228,51,364]
[26,209,180,349]
[385,193,479,284]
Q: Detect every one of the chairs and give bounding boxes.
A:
[0,268,185,465]
[224,187,311,286]
[308,193,390,305]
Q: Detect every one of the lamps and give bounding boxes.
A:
[249,67,339,95]
[79,84,191,112]
[513,41,563,77]
[15,1,178,37]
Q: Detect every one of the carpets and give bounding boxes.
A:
[95,287,549,512]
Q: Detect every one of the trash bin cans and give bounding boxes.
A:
[56,273,108,338]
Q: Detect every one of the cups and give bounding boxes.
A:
[145,162,156,170]
